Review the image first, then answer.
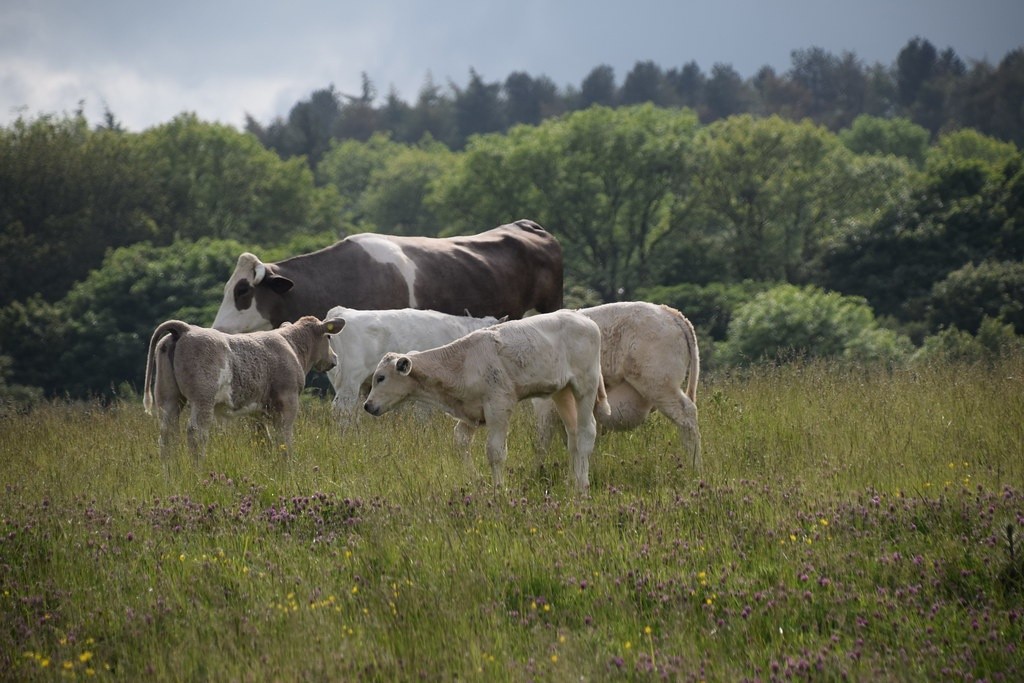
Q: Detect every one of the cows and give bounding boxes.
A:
[143,218,704,497]
